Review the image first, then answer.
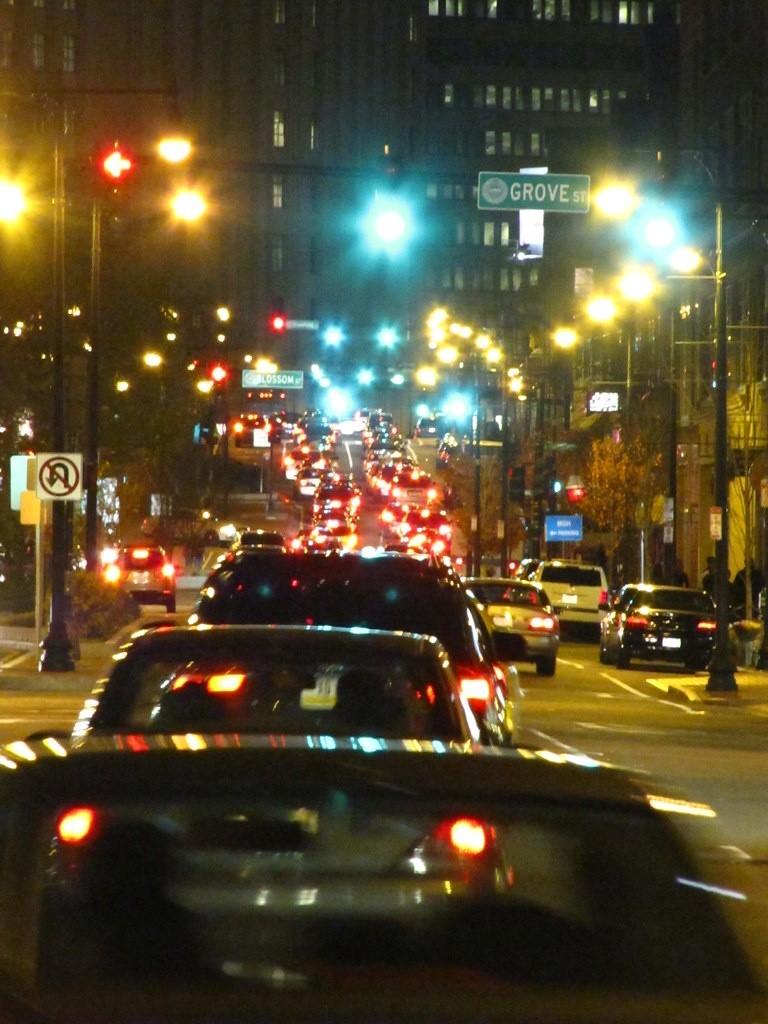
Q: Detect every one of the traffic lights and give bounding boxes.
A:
[508,463,527,500]
[92,144,134,184]
[532,458,553,501]
[208,362,229,384]
[247,392,285,404]
[270,316,285,332]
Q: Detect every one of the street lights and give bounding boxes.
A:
[417,270,633,617]
[592,177,740,691]
[80,168,207,579]
[34,84,192,671]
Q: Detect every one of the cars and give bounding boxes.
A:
[73,618,479,743]
[98,547,177,612]
[519,558,607,638]
[0,735,768,1024]
[462,577,562,678]
[600,583,717,669]
[196,544,516,743]
[230,405,499,558]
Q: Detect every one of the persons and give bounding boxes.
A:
[676,559,689,587]
[701,556,765,595]
